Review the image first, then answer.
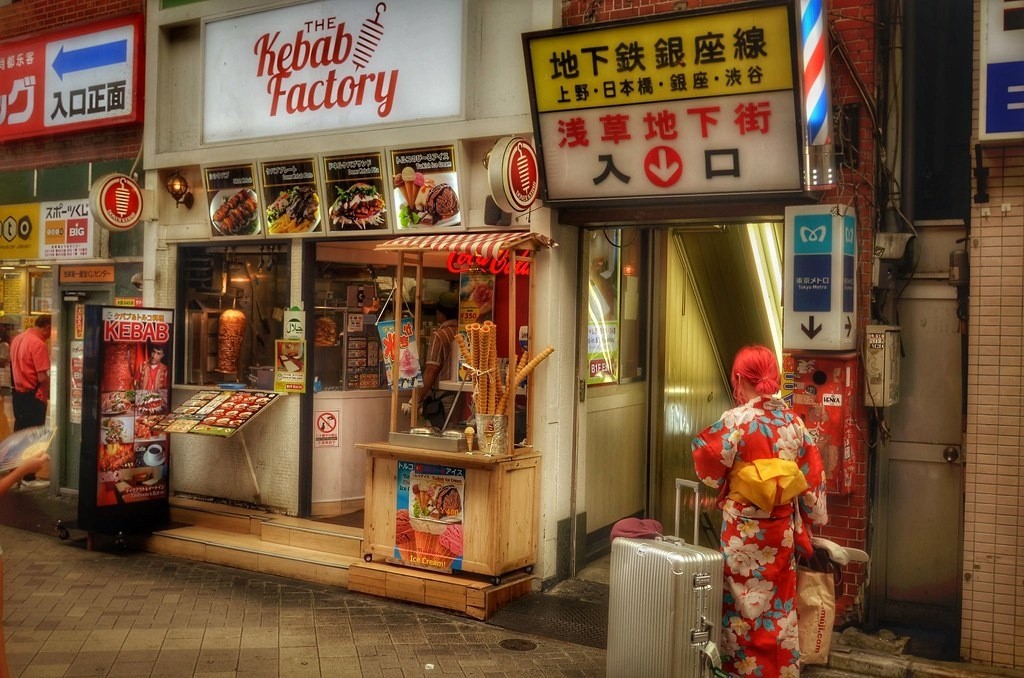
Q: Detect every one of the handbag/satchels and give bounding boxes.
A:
[795,547,844,665]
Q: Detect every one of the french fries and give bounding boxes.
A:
[267,214,311,234]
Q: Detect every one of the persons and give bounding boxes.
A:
[134,344,168,392]
[691,344,828,678]
[400,292,460,429]
[0,314,52,510]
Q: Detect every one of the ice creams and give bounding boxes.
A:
[394,167,426,210]
[395,480,464,571]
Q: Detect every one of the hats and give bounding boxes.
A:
[812,536,869,566]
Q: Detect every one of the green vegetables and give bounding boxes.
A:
[332,184,376,210]
[266,186,319,225]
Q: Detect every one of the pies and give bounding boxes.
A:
[101,392,131,413]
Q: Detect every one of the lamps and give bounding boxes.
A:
[165,169,195,211]
[481,139,497,170]
[266,252,274,272]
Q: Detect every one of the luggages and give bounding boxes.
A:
[604,477,724,678]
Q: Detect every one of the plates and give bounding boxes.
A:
[209,187,261,236]
[101,422,126,430]
[102,404,133,415]
[267,192,321,233]
[143,450,166,466]
[391,173,461,227]
[329,206,386,225]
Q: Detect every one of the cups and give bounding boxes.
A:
[146,444,163,461]
